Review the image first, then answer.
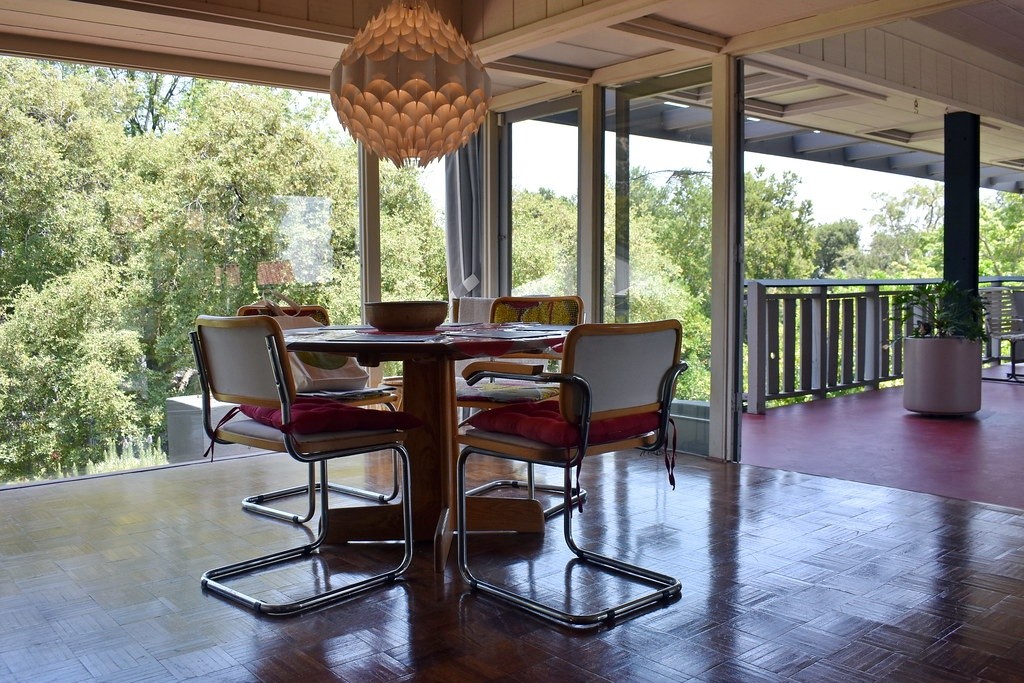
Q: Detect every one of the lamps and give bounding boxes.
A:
[329,0,493,170]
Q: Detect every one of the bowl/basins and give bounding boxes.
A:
[364,301,448,332]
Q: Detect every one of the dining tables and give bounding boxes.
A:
[271,321,578,574]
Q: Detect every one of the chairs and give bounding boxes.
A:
[978,287,1024,384]
[453,318,682,630]
[455,297,589,522]
[189,315,414,615]
[235,305,400,524]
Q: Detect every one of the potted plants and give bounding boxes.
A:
[881,278,994,417]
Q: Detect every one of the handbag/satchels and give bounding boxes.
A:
[252,289,369,392]
